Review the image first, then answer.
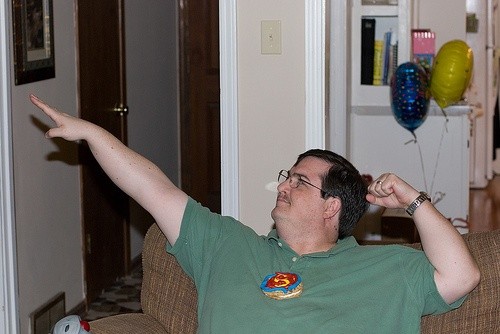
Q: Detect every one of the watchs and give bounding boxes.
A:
[405,190,432,217]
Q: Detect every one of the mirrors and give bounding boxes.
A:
[11,0,56,86]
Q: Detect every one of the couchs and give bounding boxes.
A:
[83,222,500,334]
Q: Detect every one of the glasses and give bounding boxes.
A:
[278,169,335,199]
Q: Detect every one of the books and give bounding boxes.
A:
[361,18,398,85]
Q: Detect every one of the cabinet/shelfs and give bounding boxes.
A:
[352,0,474,235]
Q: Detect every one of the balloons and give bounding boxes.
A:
[390,40,473,136]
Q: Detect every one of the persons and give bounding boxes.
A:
[28,92,482,334]
